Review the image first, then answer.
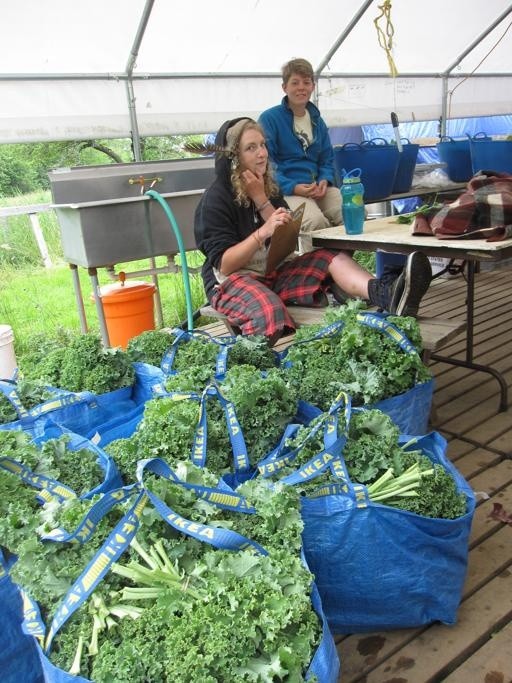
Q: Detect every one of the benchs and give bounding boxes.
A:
[199,304,469,431]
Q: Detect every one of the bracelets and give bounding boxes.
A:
[257,198,270,211]
[252,234,262,250]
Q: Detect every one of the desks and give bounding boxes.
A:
[311,201,512,411]
[366,181,480,273]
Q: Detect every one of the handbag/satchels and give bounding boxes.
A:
[0,313,475,683]
[335,132,512,200]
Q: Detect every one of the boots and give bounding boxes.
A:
[368,251,431,318]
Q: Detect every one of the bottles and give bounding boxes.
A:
[338,165,369,234]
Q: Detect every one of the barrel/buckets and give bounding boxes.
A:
[376,251,407,280]
[0,325,18,381]
[365,201,393,220]
[90,281,158,349]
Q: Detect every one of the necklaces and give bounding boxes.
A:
[252,200,258,223]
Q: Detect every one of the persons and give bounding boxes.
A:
[257,59,343,230]
[194,117,432,337]
